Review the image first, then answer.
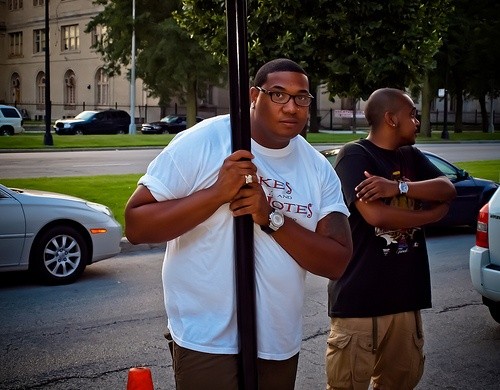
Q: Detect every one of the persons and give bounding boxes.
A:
[325,89,457,390]
[125,59,353,390]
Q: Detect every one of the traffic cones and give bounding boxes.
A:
[127,366,155,390]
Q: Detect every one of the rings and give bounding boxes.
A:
[244,175,254,184]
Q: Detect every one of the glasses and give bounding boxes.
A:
[255,85,315,107]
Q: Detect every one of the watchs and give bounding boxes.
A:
[261,208,284,235]
[397,180,409,199]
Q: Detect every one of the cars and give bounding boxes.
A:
[53,108,131,136]
[468,184,499,326]
[140,113,208,136]
[0,182,122,285]
[317,143,500,235]
[0,104,24,136]
